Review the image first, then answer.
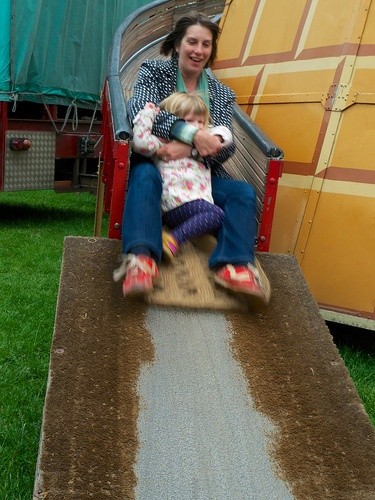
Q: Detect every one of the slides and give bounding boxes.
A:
[101,0,284,253]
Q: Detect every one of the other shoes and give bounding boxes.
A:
[161,232,181,262]
[215,264,267,305]
[112,254,158,294]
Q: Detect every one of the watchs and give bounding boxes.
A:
[191,147,199,158]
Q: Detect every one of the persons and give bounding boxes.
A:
[112,13,267,305]
[131,92,235,262]
